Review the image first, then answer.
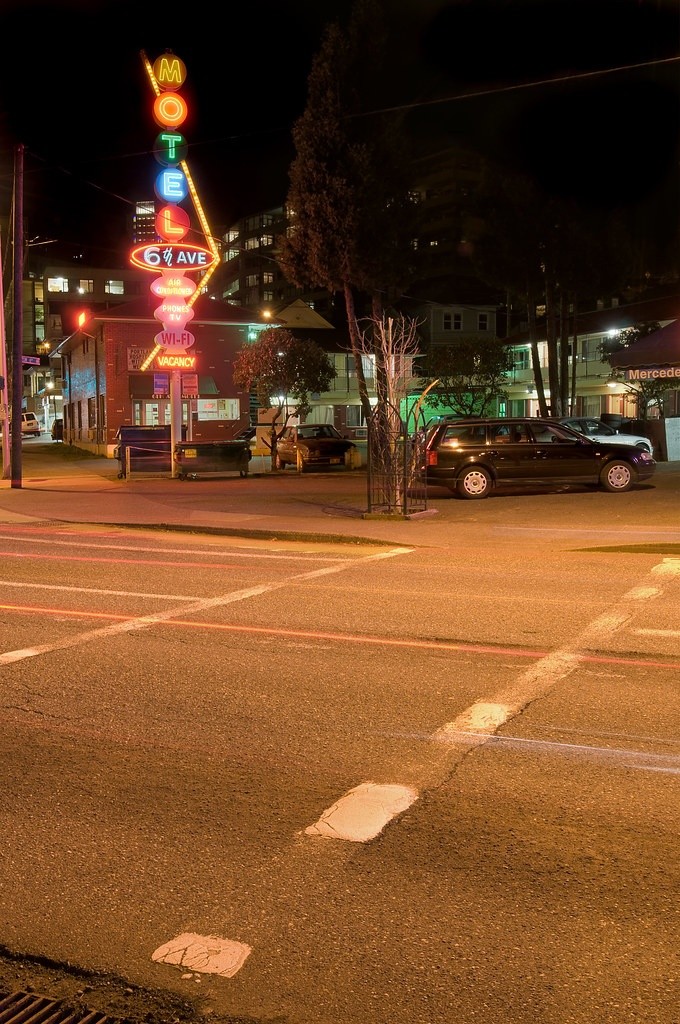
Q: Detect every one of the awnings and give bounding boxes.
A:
[611,319,680,369]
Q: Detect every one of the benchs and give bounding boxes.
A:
[113,425,253,479]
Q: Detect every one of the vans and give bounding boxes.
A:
[20,411,41,437]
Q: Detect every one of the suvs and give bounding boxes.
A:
[51,419,63,441]
[414,417,657,499]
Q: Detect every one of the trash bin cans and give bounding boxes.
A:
[345,447,361,467]
[114,424,188,479]
[51,419,63,439]
[172,439,251,479]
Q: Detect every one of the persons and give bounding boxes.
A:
[515,433,521,441]
[418,426,426,443]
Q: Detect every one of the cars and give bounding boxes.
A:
[276,423,360,473]
[525,417,654,459]
[409,415,486,454]
